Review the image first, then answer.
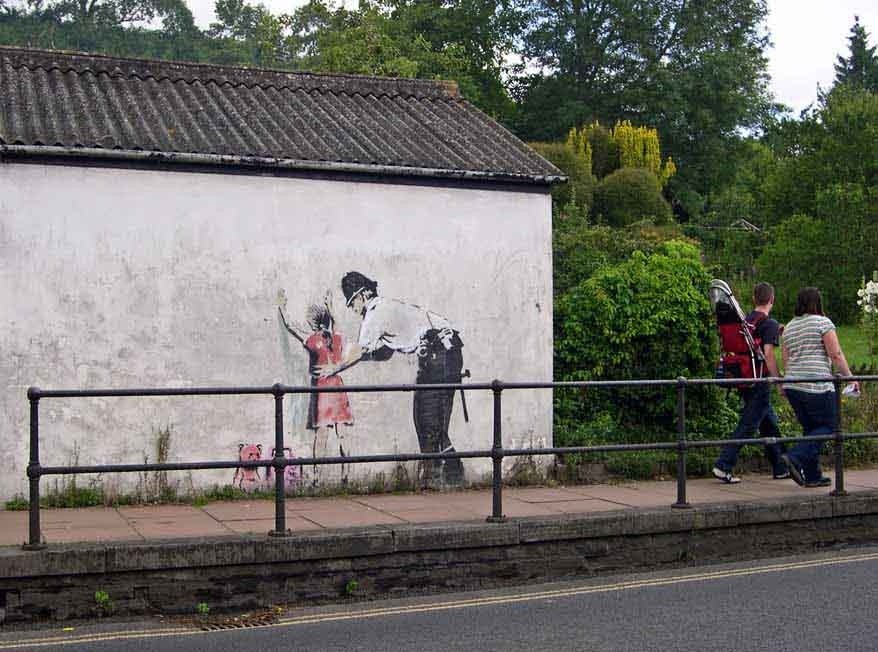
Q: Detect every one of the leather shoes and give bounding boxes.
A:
[772,466,804,480]
[712,463,741,483]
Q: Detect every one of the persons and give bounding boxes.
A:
[712,282,792,483]
[779,287,861,488]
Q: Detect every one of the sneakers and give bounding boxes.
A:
[781,453,805,487]
[806,474,832,487]
